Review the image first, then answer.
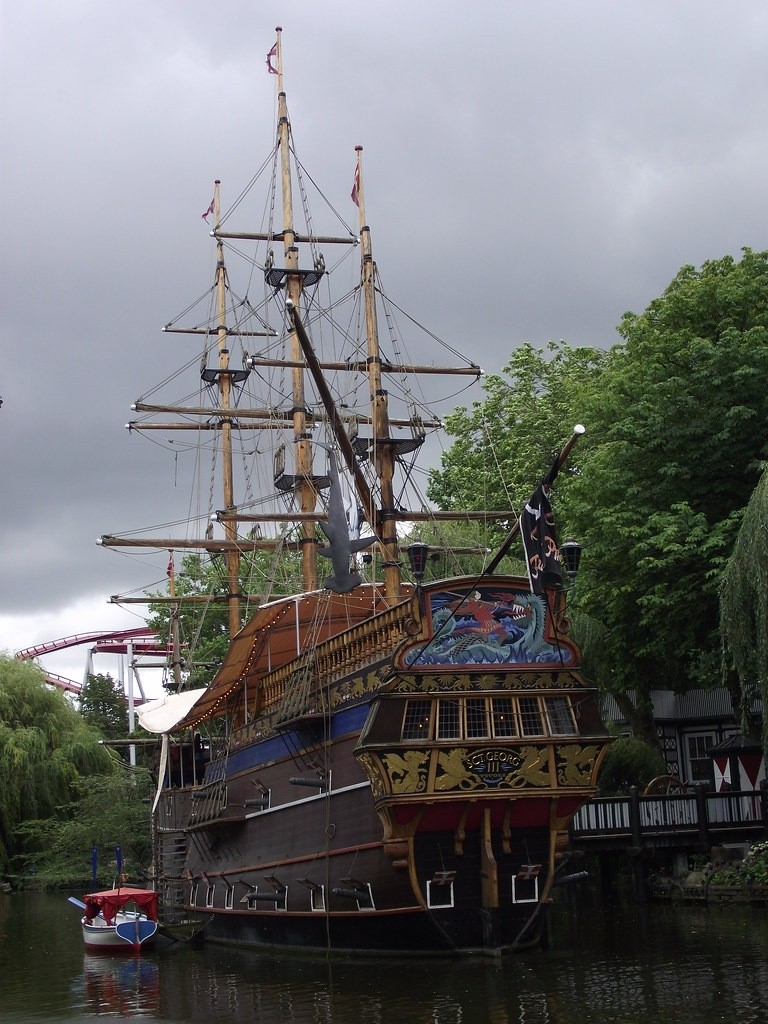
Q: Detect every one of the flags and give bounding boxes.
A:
[521,469,566,587]
[351,165,360,206]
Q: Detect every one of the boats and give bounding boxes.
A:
[67,844,162,955]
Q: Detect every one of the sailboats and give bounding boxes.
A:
[84,19,631,965]
